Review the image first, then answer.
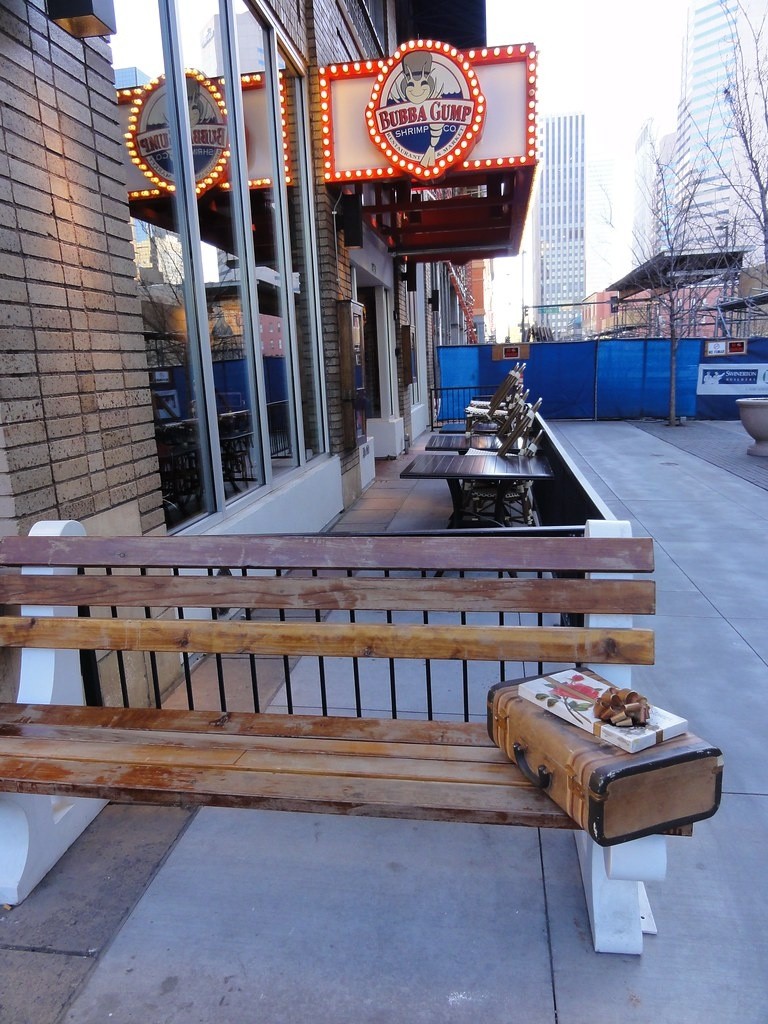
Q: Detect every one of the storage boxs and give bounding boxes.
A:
[518,670,688,753]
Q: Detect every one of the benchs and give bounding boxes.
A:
[0,520,694,956]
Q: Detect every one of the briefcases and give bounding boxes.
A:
[486,666,725,848]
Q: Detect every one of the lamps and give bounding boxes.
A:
[336,194,364,248]
[401,263,416,291]
[428,290,439,311]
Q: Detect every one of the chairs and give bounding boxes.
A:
[448,362,544,526]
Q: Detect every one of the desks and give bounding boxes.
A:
[156,431,254,517]
[399,423,555,529]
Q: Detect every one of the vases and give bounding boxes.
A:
[736,398,768,457]
